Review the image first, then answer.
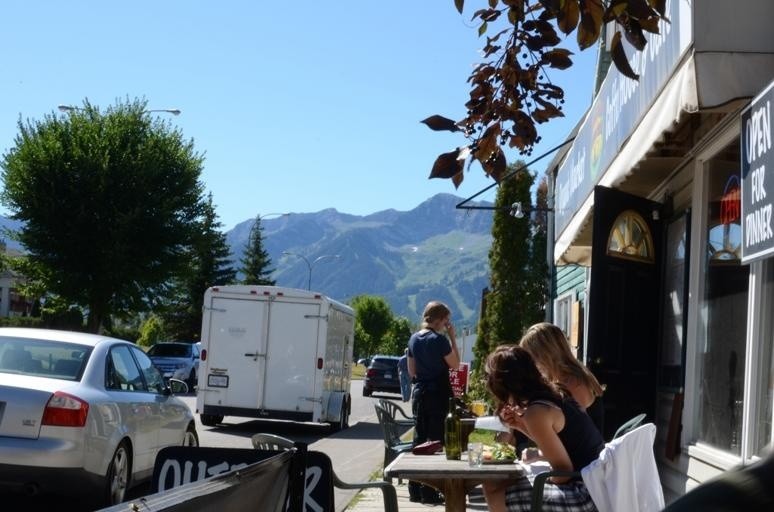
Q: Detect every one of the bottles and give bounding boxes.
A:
[444,398,462,461]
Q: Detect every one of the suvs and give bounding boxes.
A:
[363,355,400,397]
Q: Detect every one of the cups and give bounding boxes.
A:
[469,399,488,418]
[467,442,483,467]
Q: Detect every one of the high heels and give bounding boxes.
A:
[419,486,445,503]
[408,484,421,502]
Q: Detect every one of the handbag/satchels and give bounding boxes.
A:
[412,440,442,455]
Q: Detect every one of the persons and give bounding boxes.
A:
[398,348,412,402]
[519,323,614,443]
[406,302,459,503]
[483,346,605,511]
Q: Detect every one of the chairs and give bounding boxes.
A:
[374,399,421,478]
[531,423,657,512]
[250,433,398,512]
[611,413,647,442]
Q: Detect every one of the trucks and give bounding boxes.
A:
[195,284,353,432]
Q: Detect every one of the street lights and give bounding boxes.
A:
[57,101,183,122]
[245,211,291,278]
[281,249,341,291]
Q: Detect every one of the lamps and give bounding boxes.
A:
[509,200,553,219]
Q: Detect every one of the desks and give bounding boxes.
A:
[456,409,509,446]
[384,445,523,512]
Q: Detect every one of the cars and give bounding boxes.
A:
[145,341,200,389]
[0,326,200,504]
